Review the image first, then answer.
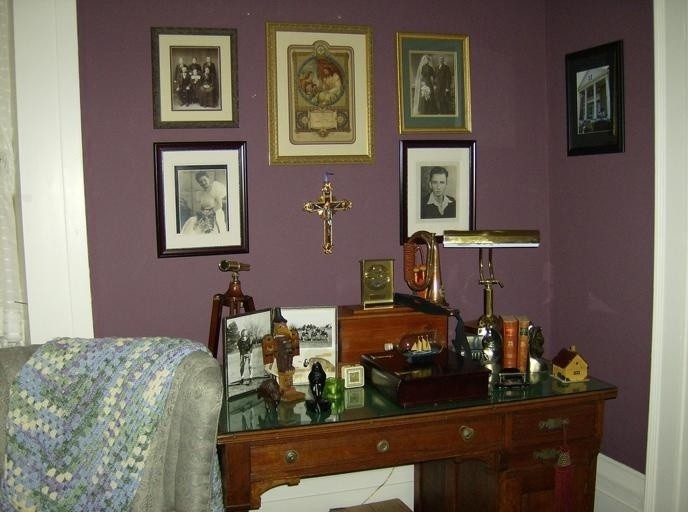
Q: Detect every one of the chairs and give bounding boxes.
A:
[0,339,223,511]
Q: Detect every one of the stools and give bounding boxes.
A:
[330,498,413,512]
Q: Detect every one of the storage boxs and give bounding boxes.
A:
[339,304,449,365]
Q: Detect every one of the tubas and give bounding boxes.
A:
[403,231,459,316]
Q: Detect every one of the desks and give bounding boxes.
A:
[216,373,617,512]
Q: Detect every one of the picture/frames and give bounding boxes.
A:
[265,22,376,166]
[153,142,249,258]
[397,30,473,133]
[151,26,240,129]
[221,309,276,401]
[399,139,478,245]
[277,306,340,384]
[565,38,627,157]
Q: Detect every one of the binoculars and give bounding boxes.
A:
[207,259,256,359]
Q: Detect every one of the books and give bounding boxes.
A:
[498,313,518,369]
[514,313,530,382]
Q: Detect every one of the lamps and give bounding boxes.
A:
[444,229,541,338]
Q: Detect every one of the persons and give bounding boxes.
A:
[236,329,254,385]
[180,171,226,234]
[414,55,439,115]
[421,166,456,219]
[580,119,587,134]
[241,403,255,431]
[300,321,329,343]
[317,66,344,106]
[172,55,217,108]
[433,56,452,115]
